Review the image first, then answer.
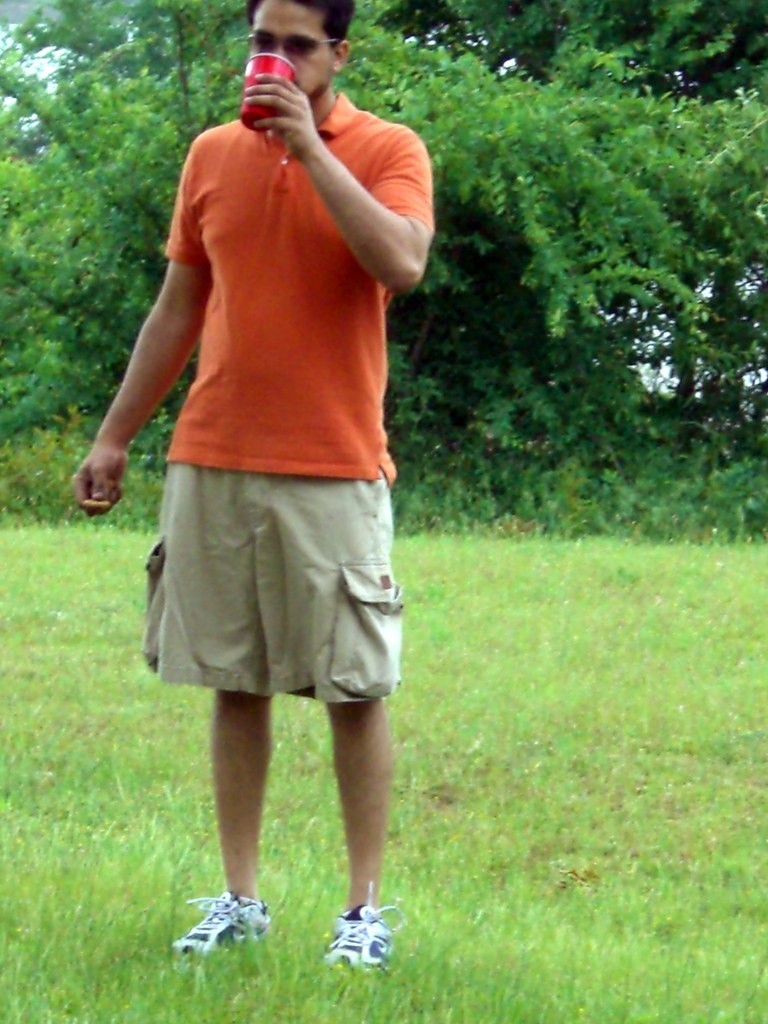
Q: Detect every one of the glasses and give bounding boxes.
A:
[248,28,342,57]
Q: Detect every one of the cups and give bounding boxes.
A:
[238,51,296,133]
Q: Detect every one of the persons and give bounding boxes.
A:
[69,0,435,973]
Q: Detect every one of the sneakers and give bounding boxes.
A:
[171,891,270,955]
[323,881,404,971]
